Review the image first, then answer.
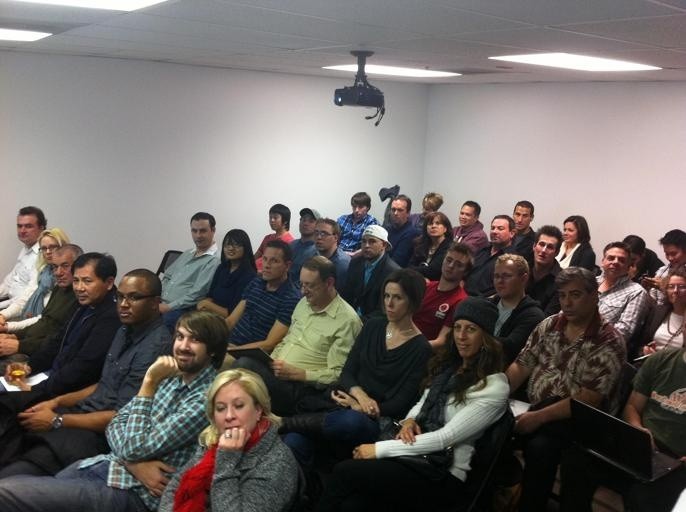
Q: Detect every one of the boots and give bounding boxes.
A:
[279,413,326,434]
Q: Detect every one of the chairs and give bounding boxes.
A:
[1,223,686,512]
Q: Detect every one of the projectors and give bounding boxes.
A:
[334,86,383,107]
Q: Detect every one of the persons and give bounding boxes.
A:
[234,193,685,512]
[1,207,307,512]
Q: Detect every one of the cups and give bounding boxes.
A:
[7,353,31,386]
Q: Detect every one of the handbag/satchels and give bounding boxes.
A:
[380,416,454,468]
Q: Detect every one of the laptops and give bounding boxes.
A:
[569,398,686,485]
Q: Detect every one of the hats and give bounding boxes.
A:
[299,208,322,220]
[452,295,500,337]
[362,225,392,252]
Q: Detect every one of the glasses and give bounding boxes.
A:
[113,293,161,303]
[39,245,58,252]
